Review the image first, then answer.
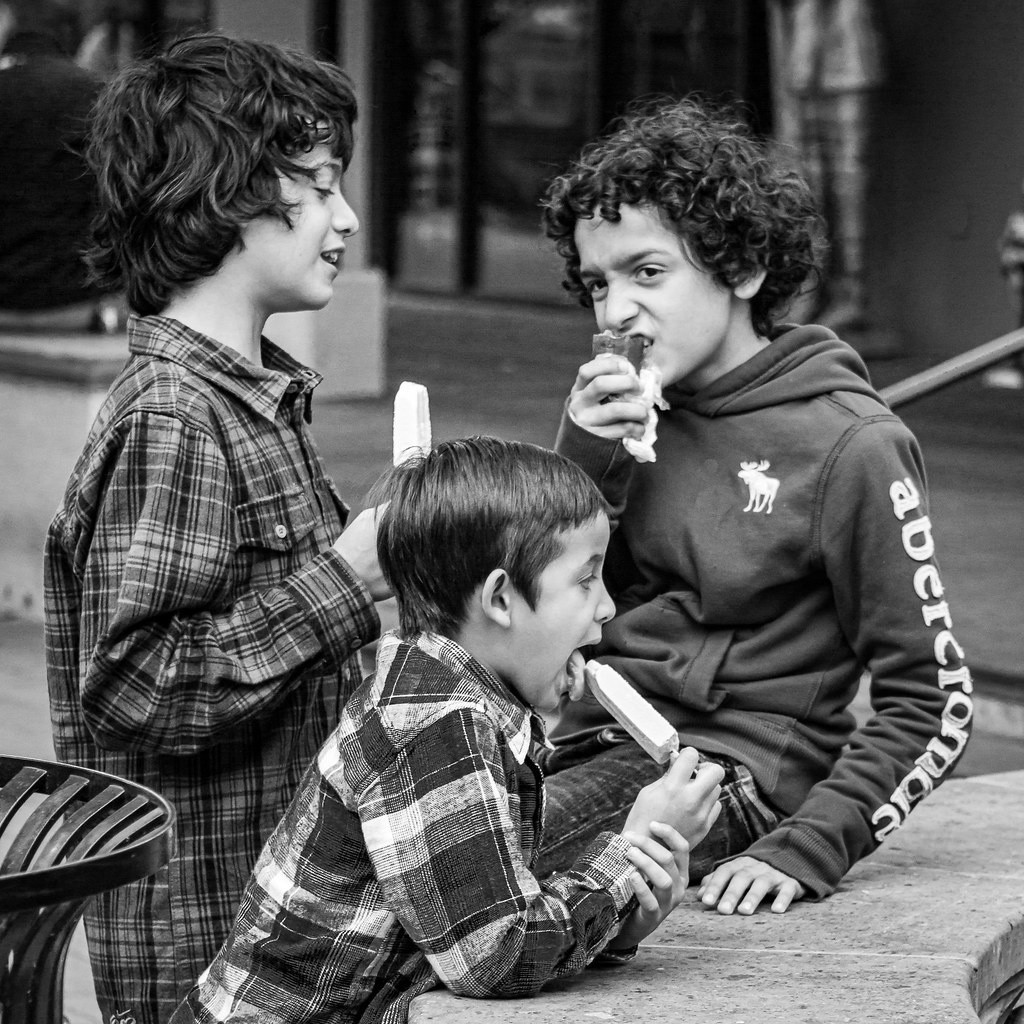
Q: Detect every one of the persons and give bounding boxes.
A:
[164,436,728,1024]
[35,30,397,1023]
[525,96,974,915]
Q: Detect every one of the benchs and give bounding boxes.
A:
[405,770,1024,1024]
